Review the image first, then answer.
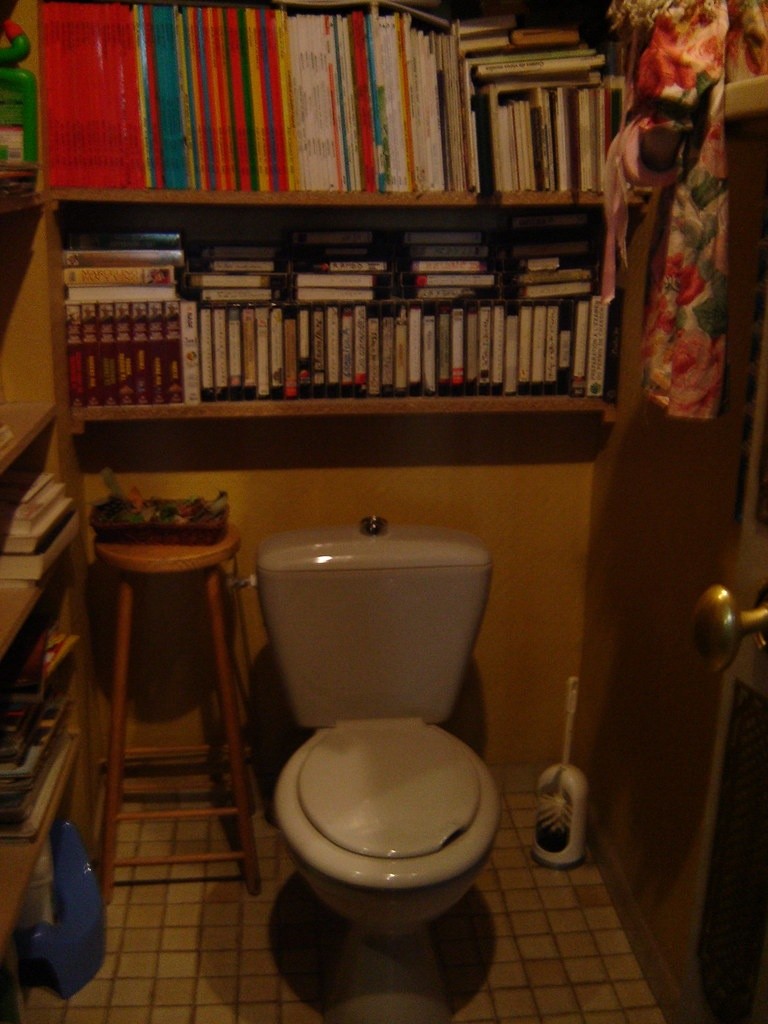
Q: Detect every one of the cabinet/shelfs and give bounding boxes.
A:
[1,183,619,1021]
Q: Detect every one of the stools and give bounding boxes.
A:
[94,530,263,897]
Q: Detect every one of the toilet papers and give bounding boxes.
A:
[601,112,683,304]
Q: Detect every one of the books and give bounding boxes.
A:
[2,464,82,579]
[2,626,78,842]
[63,219,619,404]
[131,0,628,199]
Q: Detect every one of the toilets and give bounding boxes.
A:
[257,515,502,1023]
